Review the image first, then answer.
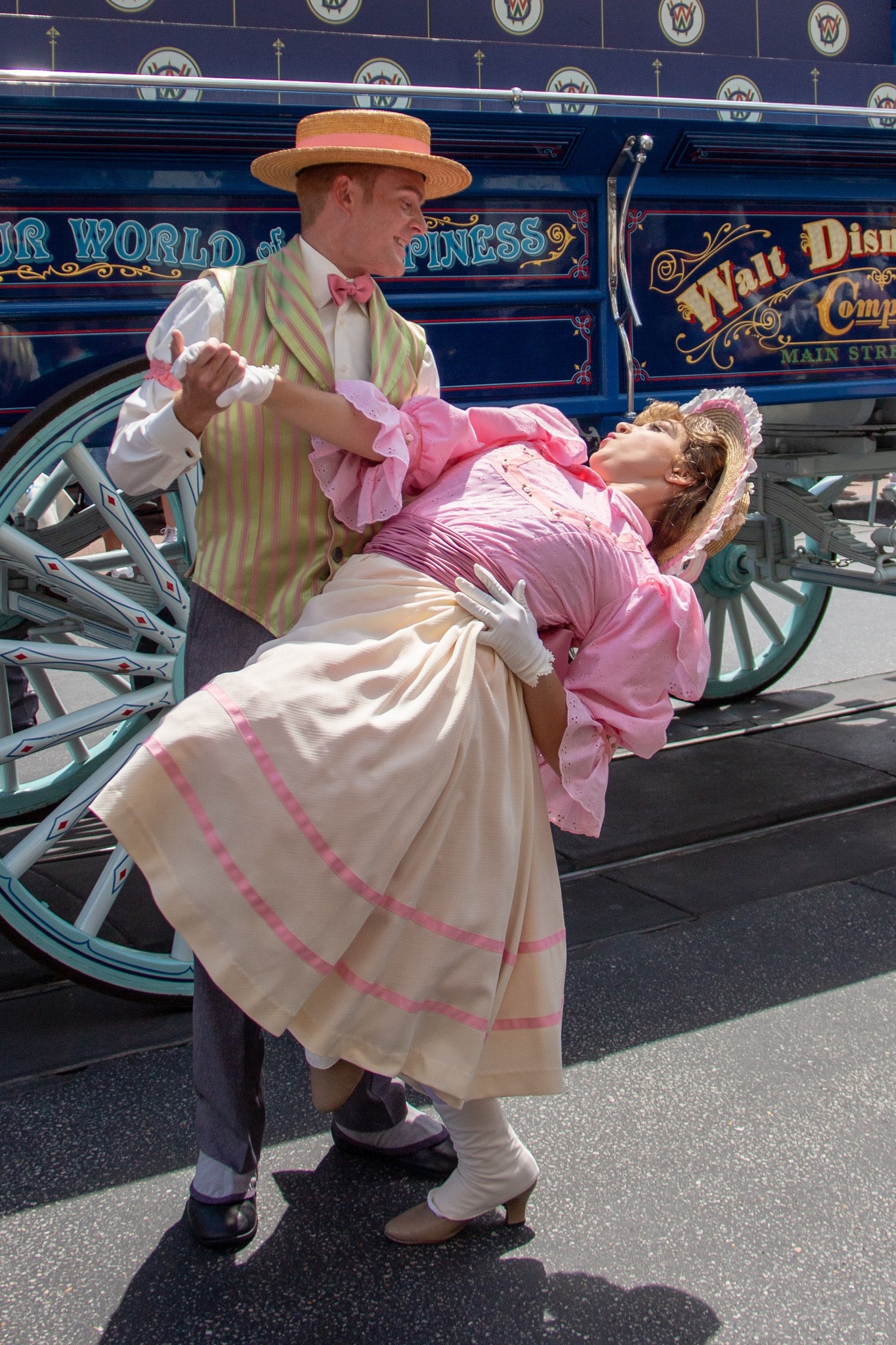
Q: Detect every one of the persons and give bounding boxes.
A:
[0,474,178,732]
[106,111,473,1246]
[91,337,763,1246]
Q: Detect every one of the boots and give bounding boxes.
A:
[383,1099,539,1246]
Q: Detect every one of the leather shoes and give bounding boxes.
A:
[331,1101,459,1173]
[186,1151,262,1246]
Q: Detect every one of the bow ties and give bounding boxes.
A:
[327,270,375,307]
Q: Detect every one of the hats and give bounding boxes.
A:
[655,384,764,583]
[251,109,473,199]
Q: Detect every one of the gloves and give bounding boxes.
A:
[454,562,556,690]
[167,340,281,407]
